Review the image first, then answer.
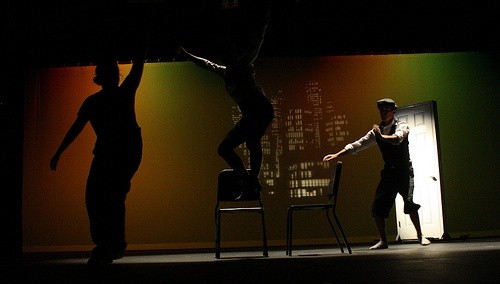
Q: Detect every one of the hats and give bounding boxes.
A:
[377,98,399,110]
[94,63,119,83]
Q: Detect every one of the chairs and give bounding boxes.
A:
[215,168,270,258]
[285,161,352,256]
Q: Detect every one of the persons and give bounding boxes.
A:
[323,98,431,250]
[50,57,151,264]
[176,10,274,201]
